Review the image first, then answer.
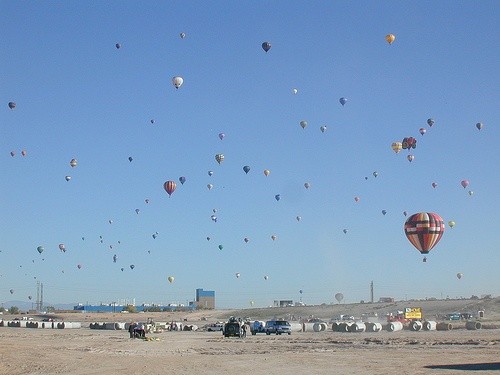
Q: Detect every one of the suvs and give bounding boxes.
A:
[223,321,247,339]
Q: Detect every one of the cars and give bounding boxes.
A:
[386,308,475,322]
[308,310,377,322]
[208,322,223,332]
[249,321,265,334]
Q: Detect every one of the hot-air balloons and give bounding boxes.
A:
[385,33,395,46]
[382,209,387,216]
[202,121,329,250]
[7,199,160,301]
[166,270,346,306]
[261,41,272,54]
[179,32,185,40]
[163,175,187,199]
[338,97,347,107]
[456,271,462,279]
[354,171,378,203]
[461,180,474,197]
[291,88,297,94]
[7,40,158,182]
[476,122,483,132]
[389,115,436,163]
[402,210,456,263]
[170,76,183,92]
[431,182,438,188]
[343,229,348,234]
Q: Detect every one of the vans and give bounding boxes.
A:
[264,321,293,335]
